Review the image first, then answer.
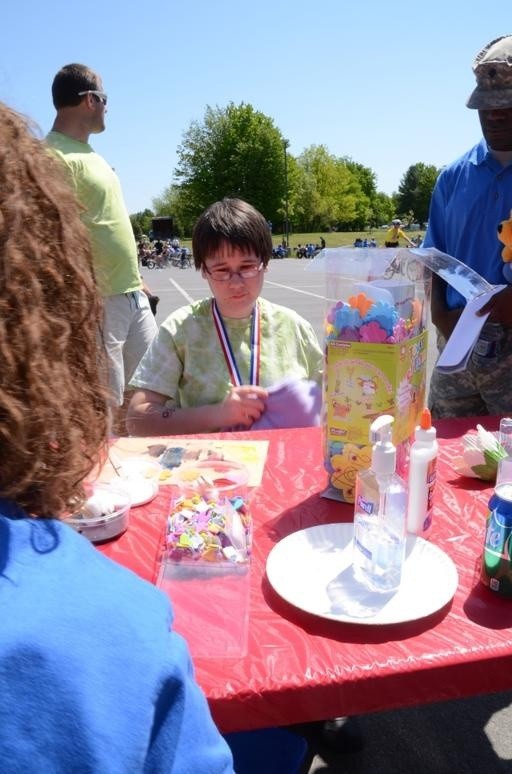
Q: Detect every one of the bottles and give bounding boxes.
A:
[407,408,440,538]
[495,416,512,486]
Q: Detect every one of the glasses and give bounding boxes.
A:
[202,259,264,281]
[79,90,108,106]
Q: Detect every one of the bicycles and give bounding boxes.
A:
[369,250,423,282]
[137,242,324,270]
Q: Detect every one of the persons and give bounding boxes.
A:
[421,36,511,424]
[37,64,158,439]
[0,104,240,772]
[136,230,193,269]
[268,219,325,259]
[354,219,422,248]
[125,196,326,438]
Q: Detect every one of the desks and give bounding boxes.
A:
[29,415,512,735]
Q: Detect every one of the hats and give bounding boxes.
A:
[467,35,511,111]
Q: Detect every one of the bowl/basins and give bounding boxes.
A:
[63,483,129,542]
[98,457,163,509]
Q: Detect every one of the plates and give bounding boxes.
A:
[265,523,459,626]
